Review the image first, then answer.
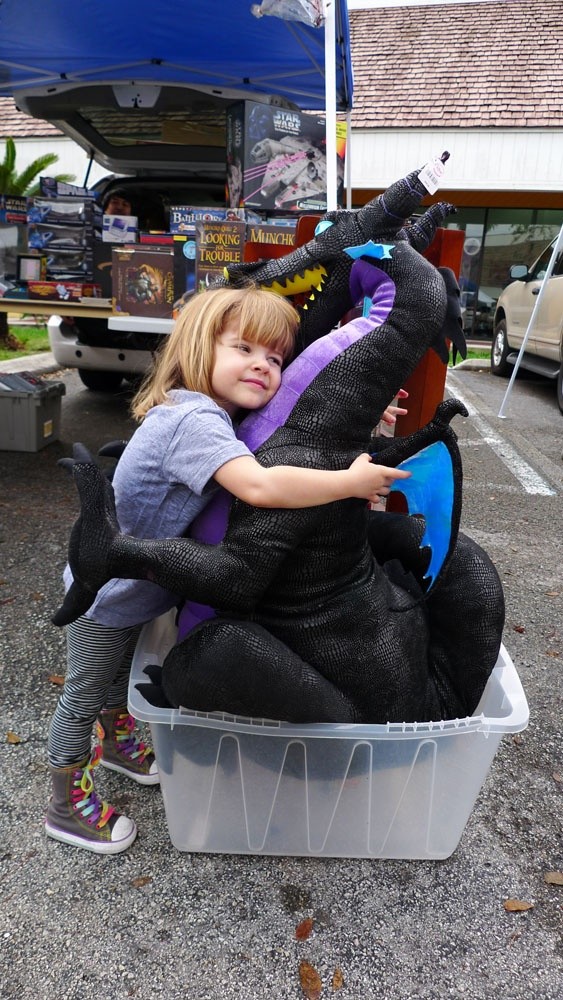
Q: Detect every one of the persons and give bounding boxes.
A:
[103,189,136,216]
[44,277,411,853]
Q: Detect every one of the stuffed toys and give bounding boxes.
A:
[52,152,506,780]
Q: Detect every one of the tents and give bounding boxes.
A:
[1,0,354,212]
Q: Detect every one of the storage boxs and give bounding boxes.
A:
[0,98,349,319]
[125,608,529,860]
[0,380,66,452]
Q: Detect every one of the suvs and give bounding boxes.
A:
[490,231,563,414]
[458,275,498,340]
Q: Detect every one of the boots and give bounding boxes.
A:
[45,744,138,854]
[98,706,161,785]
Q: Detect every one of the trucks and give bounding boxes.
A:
[0,0,353,396]
